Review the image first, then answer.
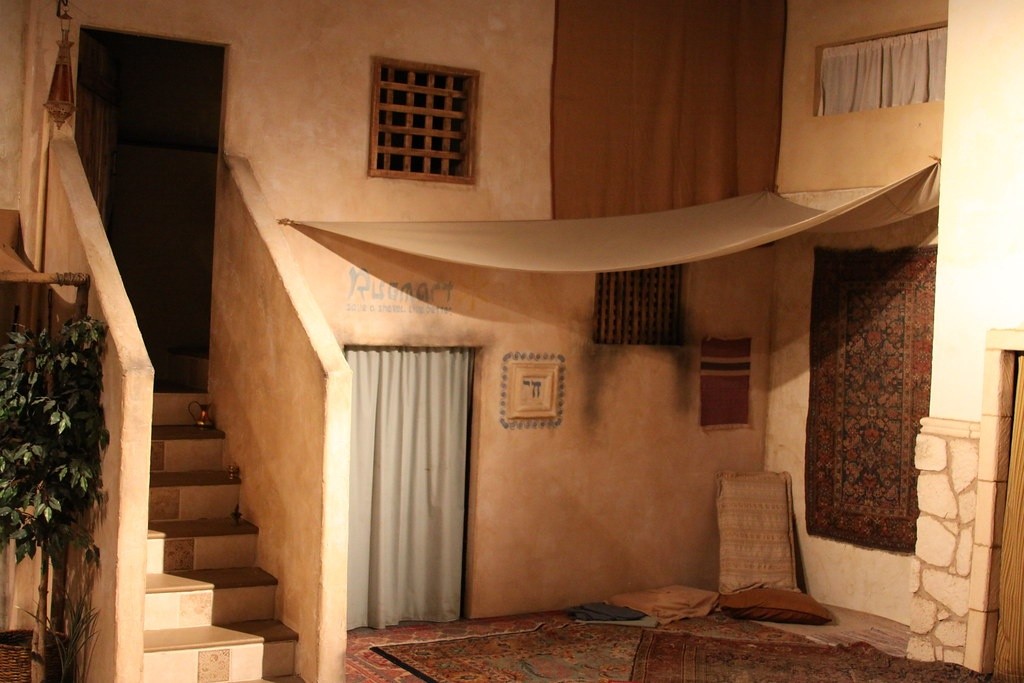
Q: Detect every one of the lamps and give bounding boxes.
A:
[42,0,77,132]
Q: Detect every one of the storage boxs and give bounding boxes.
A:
[0,628,69,683]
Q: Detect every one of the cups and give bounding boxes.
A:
[188,400,214,429]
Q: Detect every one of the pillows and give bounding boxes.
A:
[714,470,801,595]
[717,588,835,625]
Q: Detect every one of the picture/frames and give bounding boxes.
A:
[506,361,562,419]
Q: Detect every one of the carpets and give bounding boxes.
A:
[629,627,994,683]
[369,620,644,682]
